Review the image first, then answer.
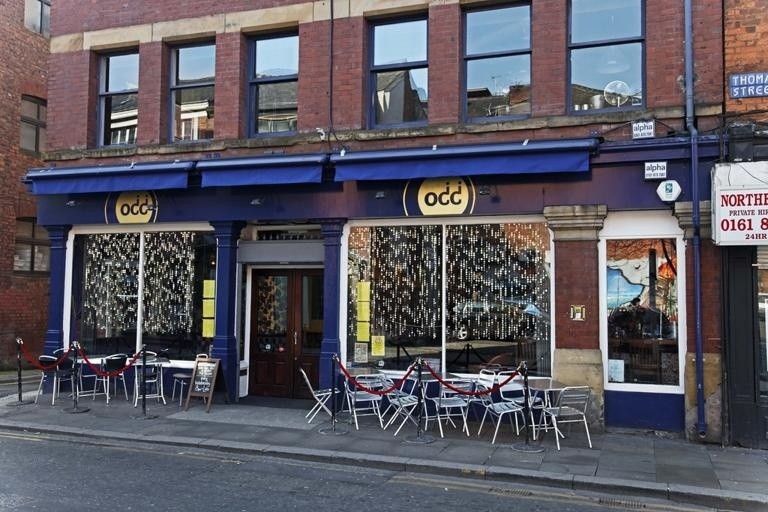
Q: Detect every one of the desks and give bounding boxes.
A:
[77,357,196,400]
[342,366,384,425]
[513,379,568,441]
[410,372,460,431]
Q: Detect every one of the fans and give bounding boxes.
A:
[603,80,629,107]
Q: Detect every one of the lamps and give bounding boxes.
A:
[250,198,262,208]
[478,188,491,196]
[375,191,387,199]
[65,200,79,209]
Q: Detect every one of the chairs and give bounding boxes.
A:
[445,369,495,432]
[92,354,129,405]
[132,351,167,408]
[477,383,526,445]
[498,371,549,436]
[348,375,385,430]
[172,354,208,407]
[380,377,424,436]
[53,347,74,399]
[34,355,71,406]
[430,380,473,438]
[298,367,341,423]
[536,385,594,450]
[380,375,417,425]
